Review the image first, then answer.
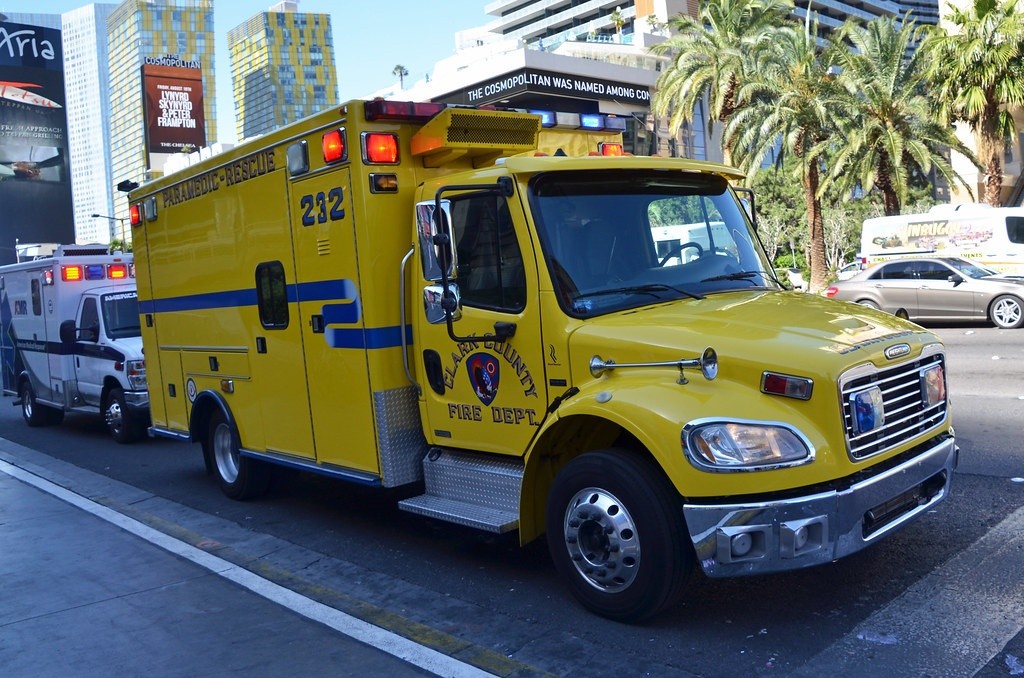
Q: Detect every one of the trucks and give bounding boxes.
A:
[859,201,1024,281]
[0,245,152,444]
[119,94,958,628]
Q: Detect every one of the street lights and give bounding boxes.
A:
[14,237,21,262]
[91,213,130,253]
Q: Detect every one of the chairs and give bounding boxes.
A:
[579,220,617,279]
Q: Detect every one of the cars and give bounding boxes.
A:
[827,259,863,281]
[774,265,803,288]
[823,256,1024,334]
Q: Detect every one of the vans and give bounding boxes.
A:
[649,221,740,268]
